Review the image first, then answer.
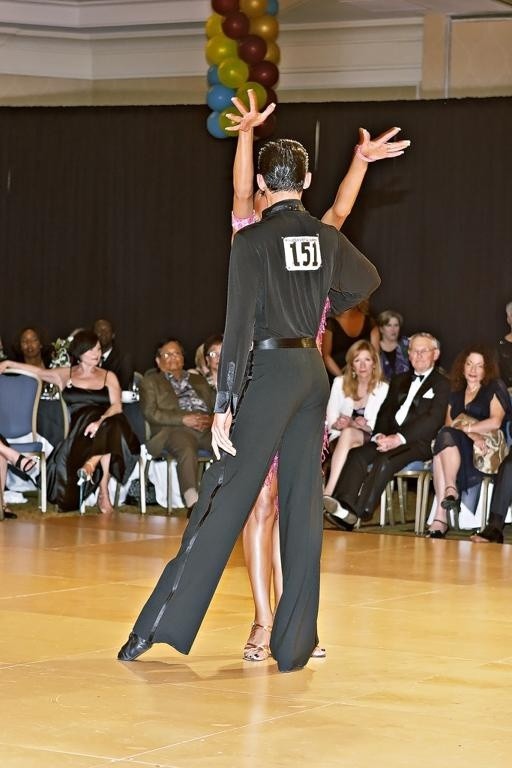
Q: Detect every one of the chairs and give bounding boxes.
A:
[354,459,510,535]
[0,369,213,518]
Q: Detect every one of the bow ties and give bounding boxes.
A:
[411,373,425,382]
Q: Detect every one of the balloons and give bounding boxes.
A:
[206,0,280,141]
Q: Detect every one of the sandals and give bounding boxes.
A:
[242,620,273,662]
[2,505,17,519]
[15,454,37,472]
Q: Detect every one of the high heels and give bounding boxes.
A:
[426,520,449,538]
[77,461,95,486]
[97,494,115,514]
[441,486,461,512]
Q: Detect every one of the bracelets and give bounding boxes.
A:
[354,144,376,162]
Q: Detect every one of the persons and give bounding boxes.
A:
[225,90,411,663]
[118,138,381,672]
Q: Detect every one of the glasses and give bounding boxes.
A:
[160,351,182,359]
[206,351,219,358]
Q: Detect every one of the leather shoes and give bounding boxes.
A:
[470,526,503,544]
[118,631,152,661]
[322,495,358,532]
